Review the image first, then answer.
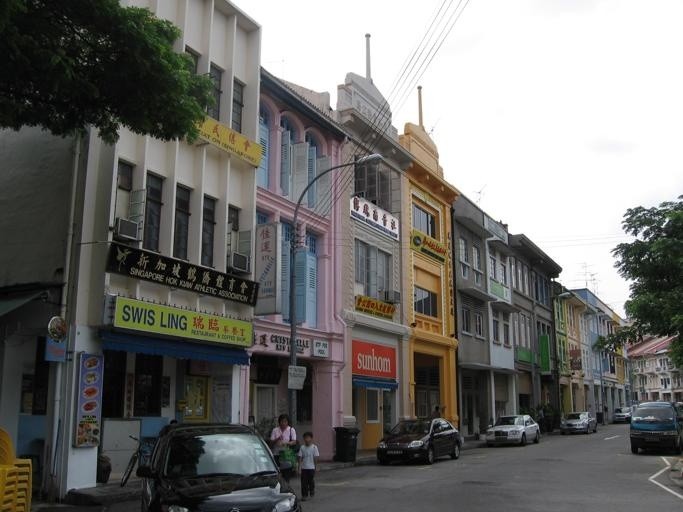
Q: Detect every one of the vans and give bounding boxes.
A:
[630,402,683,455]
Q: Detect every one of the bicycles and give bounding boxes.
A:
[120,435,155,487]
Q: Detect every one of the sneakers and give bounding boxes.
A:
[300,491,317,501]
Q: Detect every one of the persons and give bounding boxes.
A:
[432,405,440,418]
[269,413,296,473]
[296,431,319,501]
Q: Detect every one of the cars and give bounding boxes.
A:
[376,406,632,464]
[137,422,302,512]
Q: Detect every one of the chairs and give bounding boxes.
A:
[0,428,33,512]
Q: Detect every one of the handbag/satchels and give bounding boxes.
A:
[289,427,301,455]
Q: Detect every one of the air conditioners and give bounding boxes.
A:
[227,251,248,273]
[115,217,138,243]
[385,289,401,304]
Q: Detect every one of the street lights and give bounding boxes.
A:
[288,153,384,426]
[531,291,572,411]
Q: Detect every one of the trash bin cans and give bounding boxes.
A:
[596,412,603,423]
[332,426,360,462]
